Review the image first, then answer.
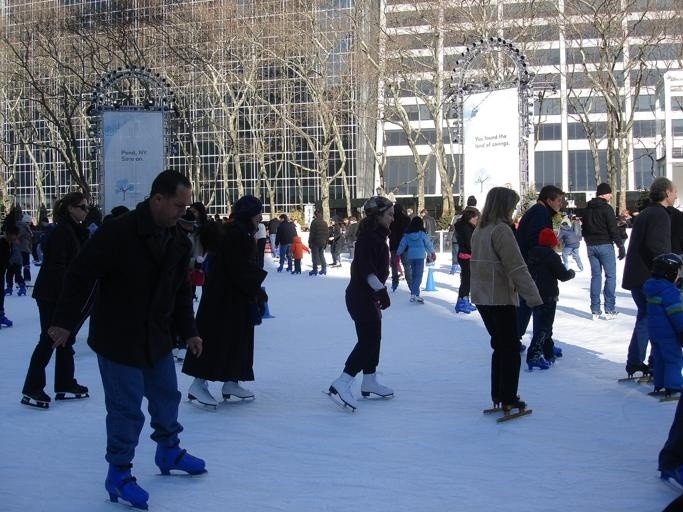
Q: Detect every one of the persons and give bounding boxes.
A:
[657,391,683,512]
[581,183,626,315]
[322,196,395,409]
[622,177,677,374]
[1,199,683,328]
[21,192,89,403]
[471,187,544,411]
[182,195,263,406]
[526,228,575,368]
[516,185,563,351]
[46,170,205,504]
[641,252,683,393]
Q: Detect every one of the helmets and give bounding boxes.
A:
[651,253,683,278]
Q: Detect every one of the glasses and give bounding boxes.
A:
[76,204,88,211]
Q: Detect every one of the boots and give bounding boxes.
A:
[54,379,88,394]
[106,465,149,503]
[222,380,255,397]
[188,378,218,405]
[156,442,204,472]
[450,265,461,274]
[23,388,51,403]
[331,371,357,409]
[361,373,394,395]
[455,297,477,313]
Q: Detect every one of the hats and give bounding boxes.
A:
[365,197,403,216]
[597,183,612,196]
[412,217,423,226]
[178,210,195,222]
[23,215,32,222]
[236,196,262,212]
[538,228,557,246]
[467,195,476,206]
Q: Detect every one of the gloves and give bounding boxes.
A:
[617,246,625,260]
[254,224,267,239]
[376,286,390,309]
[431,252,436,260]
[394,256,400,264]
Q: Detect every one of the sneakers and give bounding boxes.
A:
[492,396,526,410]
[278,260,341,274]
[5,287,26,294]
[410,295,424,302]
[626,356,683,393]
[1,319,12,325]
[592,308,618,314]
[659,452,682,485]
[520,344,561,367]
